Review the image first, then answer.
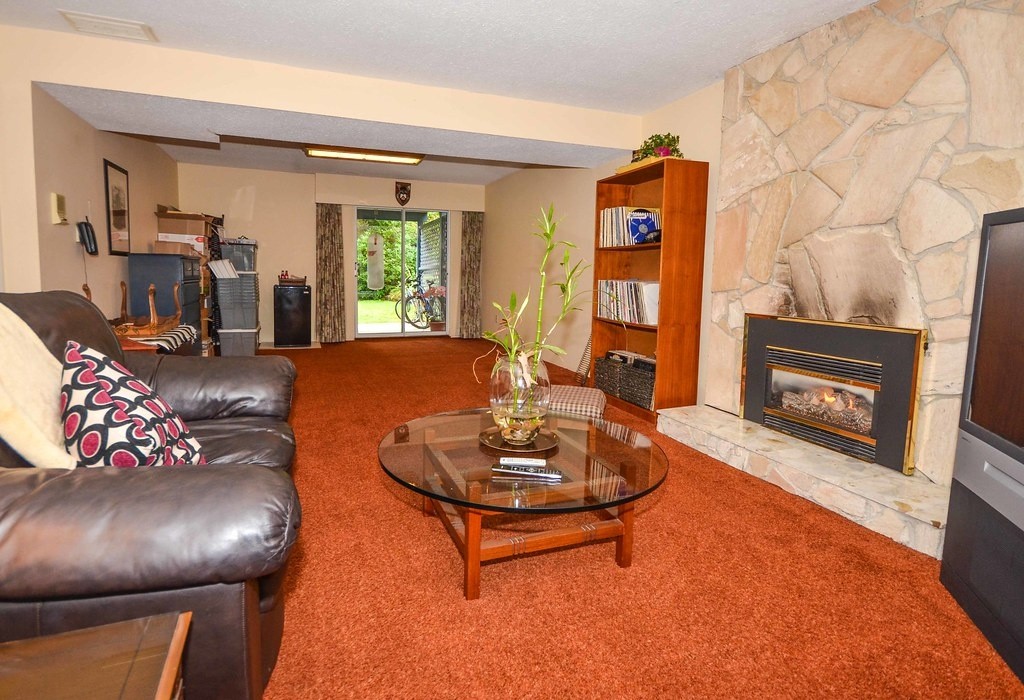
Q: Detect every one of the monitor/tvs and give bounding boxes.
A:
[959,207,1024,464]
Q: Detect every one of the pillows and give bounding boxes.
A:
[59,341,208,470]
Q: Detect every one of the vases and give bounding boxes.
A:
[428,322,446,331]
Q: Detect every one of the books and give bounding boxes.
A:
[598,206,662,248]
[594,350,656,411]
[596,418,651,448]
[598,279,659,324]
[590,458,627,509]
[208,259,240,278]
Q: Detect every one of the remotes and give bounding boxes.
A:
[491,464,562,479]
[500,457,546,467]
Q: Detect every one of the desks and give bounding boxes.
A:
[82,280,182,336]
[112,325,197,355]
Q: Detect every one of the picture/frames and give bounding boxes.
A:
[103,158,130,256]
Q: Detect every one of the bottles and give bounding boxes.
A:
[281,270,288,279]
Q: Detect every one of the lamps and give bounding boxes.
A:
[302,146,427,165]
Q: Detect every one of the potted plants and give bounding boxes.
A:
[480,202,634,449]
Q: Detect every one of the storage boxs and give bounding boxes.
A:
[215,237,261,357]
[154,204,215,237]
[155,241,191,253]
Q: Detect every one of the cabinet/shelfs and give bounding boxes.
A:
[128,252,202,355]
[594,159,710,409]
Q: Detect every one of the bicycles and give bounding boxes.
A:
[394,267,446,329]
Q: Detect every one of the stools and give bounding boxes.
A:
[498,383,606,419]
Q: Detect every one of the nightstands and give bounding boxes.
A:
[0,609,191,700]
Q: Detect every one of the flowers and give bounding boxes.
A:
[639,132,685,159]
[412,284,447,321]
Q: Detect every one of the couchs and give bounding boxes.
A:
[0,290,305,700]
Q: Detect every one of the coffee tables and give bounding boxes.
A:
[378,408,669,600]
